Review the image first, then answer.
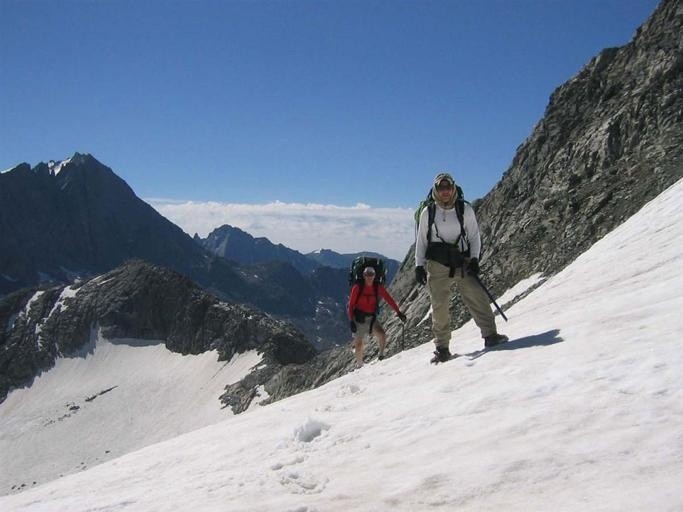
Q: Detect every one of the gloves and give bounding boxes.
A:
[399,313,406,321]
[351,321,357,332]
[415,266,427,285]
[467,257,480,277]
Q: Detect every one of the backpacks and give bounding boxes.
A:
[348,256,387,286]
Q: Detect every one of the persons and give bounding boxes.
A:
[414,173,509,364]
[346,267,407,368]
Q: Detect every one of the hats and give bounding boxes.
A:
[364,267,375,276]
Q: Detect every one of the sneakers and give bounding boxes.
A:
[431,348,451,362]
[485,334,509,348]
